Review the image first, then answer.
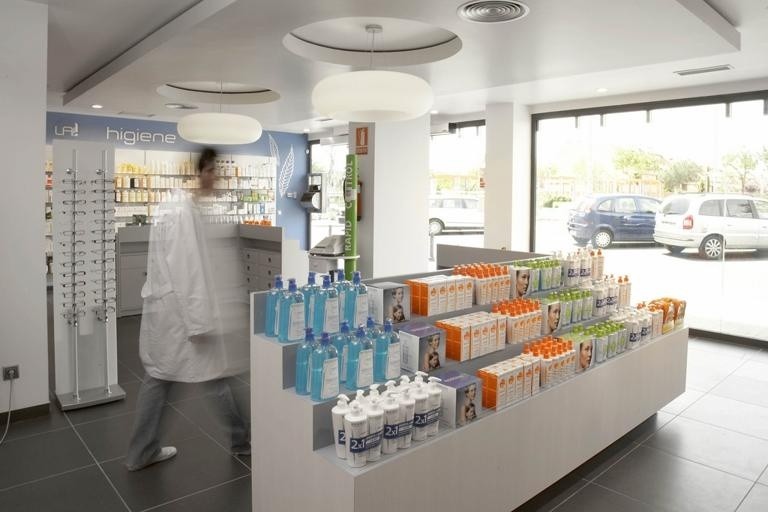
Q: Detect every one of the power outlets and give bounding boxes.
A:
[1,363,22,384]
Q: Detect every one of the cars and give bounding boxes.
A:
[337,204,344,224]
[563,193,670,251]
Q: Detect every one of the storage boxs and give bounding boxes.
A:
[478,354,540,413]
[437,310,506,363]
[366,281,409,329]
[406,275,474,318]
[430,371,482,429]
[399,323,446,375]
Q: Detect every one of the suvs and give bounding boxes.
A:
[427,191,485,236]
[653,189,768,261]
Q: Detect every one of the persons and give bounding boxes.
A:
[120,144,252,471]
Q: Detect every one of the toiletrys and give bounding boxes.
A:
[265,242,690,471]
[116,157,278,228]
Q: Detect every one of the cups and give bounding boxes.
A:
[114,176,151,189]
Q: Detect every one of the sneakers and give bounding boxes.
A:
[125,445,178,475]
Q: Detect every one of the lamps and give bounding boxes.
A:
[173,79,264,150]
[308,22,436,127]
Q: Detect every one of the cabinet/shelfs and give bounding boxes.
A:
[249,253,691,512]
[114,171,277,224]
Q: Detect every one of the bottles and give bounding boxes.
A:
[522,334,577,390]
[205,216,272,227]
[115,189,170,204]
[453,261,511,305]
[215,159,236,177]
[491,297,542,344]
